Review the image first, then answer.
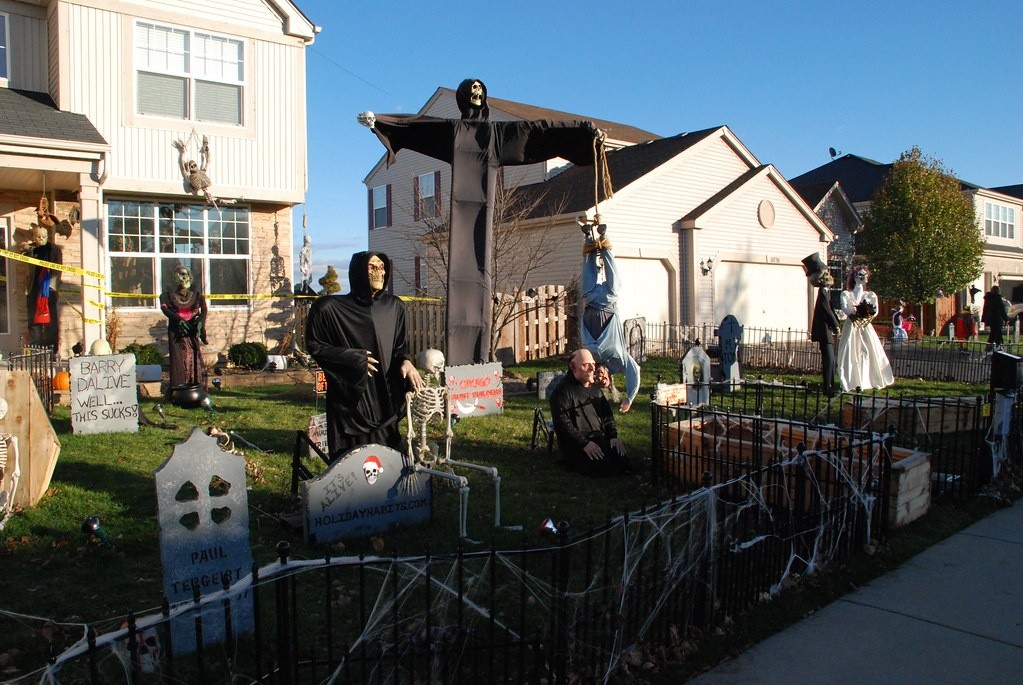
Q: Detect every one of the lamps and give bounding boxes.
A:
[700,255,714,276]
[992,271,1002,287]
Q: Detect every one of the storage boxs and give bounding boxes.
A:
[843,396,981,436]
[664,417,932,529]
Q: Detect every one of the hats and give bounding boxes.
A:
[800,252,827,276]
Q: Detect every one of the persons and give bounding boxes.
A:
[548,348,628,480]
[21,226,63,362]
[981,285,1010,352]
[578,224,640,413]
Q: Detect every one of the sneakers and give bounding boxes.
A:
[597,223,607,235]
[580,224,591,235]
